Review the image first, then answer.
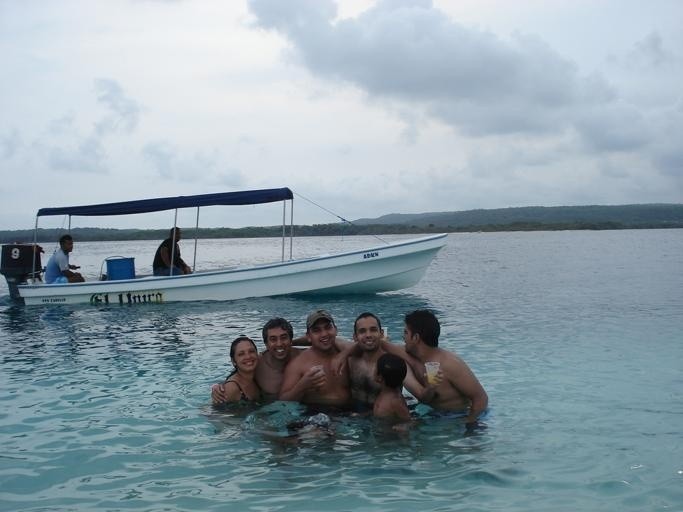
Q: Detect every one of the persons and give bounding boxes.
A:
[259,311,444,416]
[348,352,412,433]
[43,233,86,283]
[210,317,306,407]
[277,309,351,407]
[218,336,261,404]
[328,308,488,428]
[150,226,192,276]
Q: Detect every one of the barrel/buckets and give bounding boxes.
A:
[105,256,135,280]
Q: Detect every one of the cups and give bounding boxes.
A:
[425,362,440,384]
[312,365,325,385]
[380,327,387,341]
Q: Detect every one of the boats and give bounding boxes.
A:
[0,187,449,305]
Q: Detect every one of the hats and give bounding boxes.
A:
[306,309,334,328]
